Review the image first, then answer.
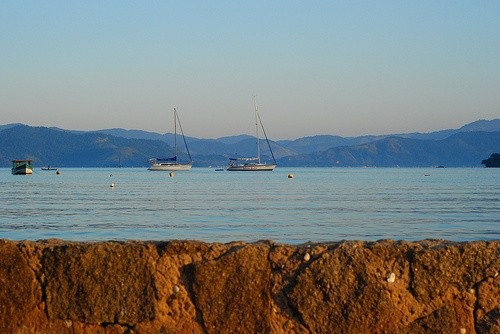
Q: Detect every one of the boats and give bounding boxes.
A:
[10,160,33,175]
[481,153,500,168]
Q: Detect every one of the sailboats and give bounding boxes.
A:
[226,95,278,172]
[147,108,194,172]
